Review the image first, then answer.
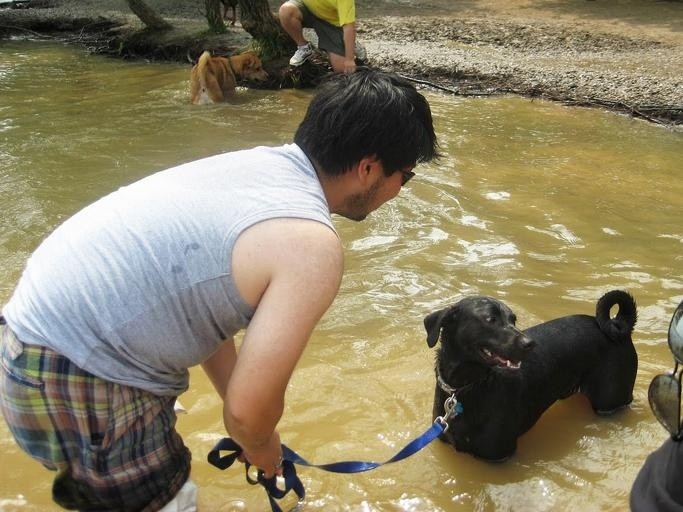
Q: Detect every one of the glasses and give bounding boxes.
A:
[393,166,416,186]
[647,296,683,446]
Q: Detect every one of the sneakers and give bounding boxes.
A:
[289,42,315,67]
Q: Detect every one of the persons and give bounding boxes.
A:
[1,69,441,511]
[278,1,358,74]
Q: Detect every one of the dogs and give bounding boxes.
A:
[188,50,270,105]
[422,289,639,464]
